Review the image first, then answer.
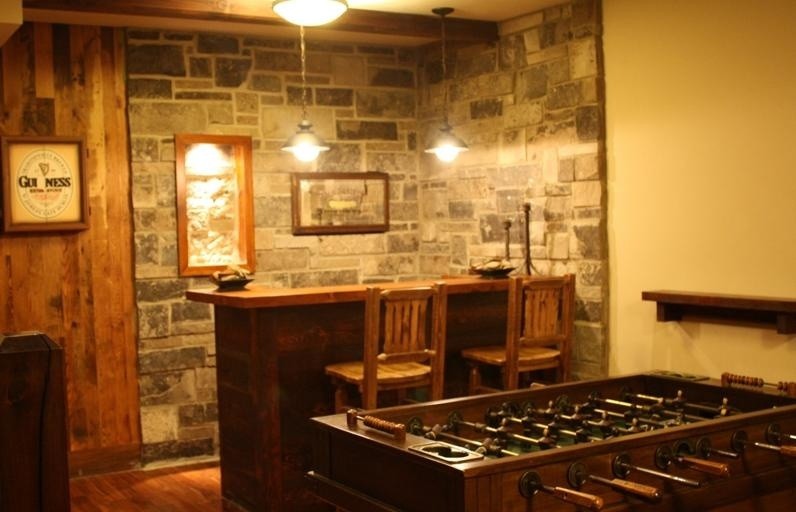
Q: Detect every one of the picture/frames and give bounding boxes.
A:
[174,135,256,277]
[2,135,91,234]
[290,172,390,233]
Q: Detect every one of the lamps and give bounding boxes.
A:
[273,0,348,27]
[425,6,470,163]
[281,26,330,162]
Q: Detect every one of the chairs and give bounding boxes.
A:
[461,274,575,396]
[324,280,449,414]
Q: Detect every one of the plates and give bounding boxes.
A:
[208,279,254,288]
[471,267,516,276]
